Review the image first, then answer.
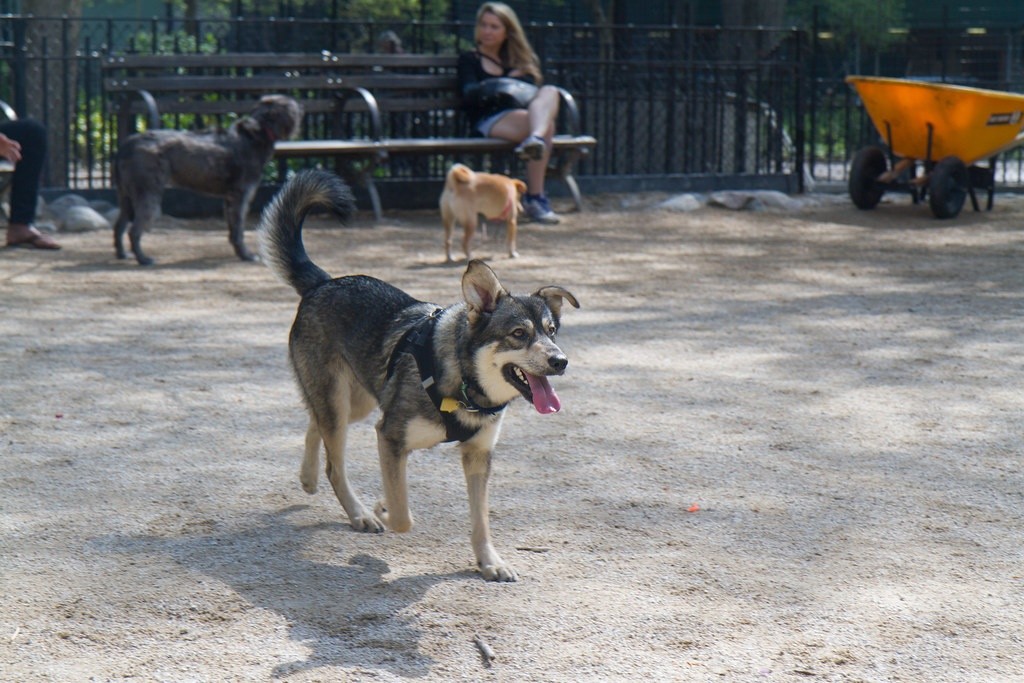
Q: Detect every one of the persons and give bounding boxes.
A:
[0,117,62,250]
[455,3,561,225]
[378,31,415,72]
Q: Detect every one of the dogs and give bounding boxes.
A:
[110,92,302,268]
[438,162,529,265]
[253,166,582,585]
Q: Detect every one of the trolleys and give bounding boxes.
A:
[846,75,1024,221]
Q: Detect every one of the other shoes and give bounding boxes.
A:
[7,224,61,250]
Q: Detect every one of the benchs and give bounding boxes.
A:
[99,54,597,222]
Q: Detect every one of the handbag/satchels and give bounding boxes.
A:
[464,78,538,122]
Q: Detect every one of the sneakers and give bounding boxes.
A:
[521,194,561,225]
[513,137,547,163]
